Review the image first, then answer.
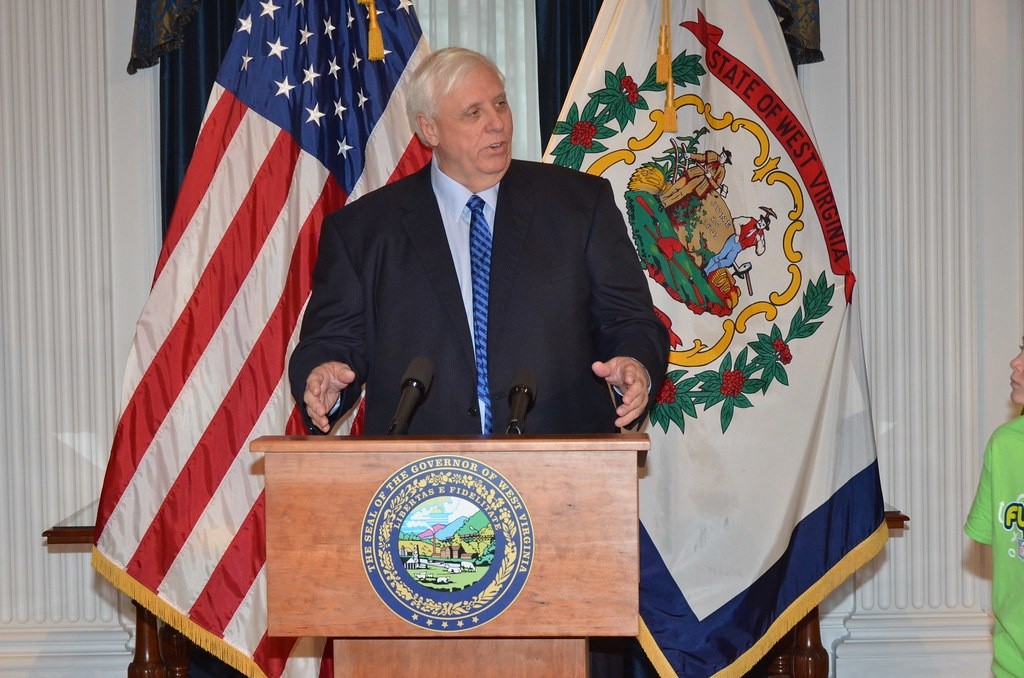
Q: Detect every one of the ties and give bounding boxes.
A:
[466,195,493,435]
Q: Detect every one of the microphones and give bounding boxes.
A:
[505,368,538,435]
[389,356,433,436]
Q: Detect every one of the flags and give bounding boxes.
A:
[94,0,439,678]
[536,0,885,678]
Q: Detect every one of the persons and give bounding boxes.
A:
[964,334,1024,678]
[288,49,670,437]
